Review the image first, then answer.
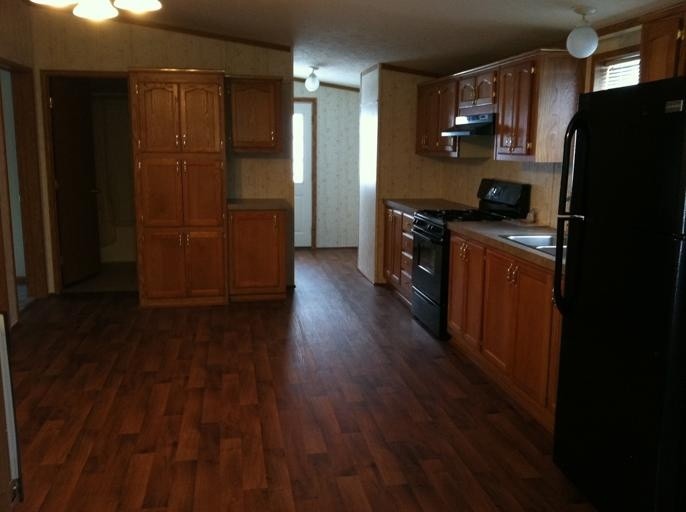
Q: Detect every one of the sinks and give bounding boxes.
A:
[507,233,569,246]
[536,244,567,261]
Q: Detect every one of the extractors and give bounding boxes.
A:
[439,113,497,143]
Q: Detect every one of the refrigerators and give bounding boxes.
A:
[551,76,686,511]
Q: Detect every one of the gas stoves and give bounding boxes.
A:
[416,208,513,224]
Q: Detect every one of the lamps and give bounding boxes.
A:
[566,23,599,58]
[304,74,320,97]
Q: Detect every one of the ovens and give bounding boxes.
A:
[411,213,448,338]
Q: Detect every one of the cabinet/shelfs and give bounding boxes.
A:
[448,237,482,353]
[477,252,547,405]
[497,56,577,167]
[459,73,493,113]
[640,18,661,82]
[546,273,570,430]
[126,70,228,307]
[228,206,294,305]
[381,211,412,303]
[226,77,284,156]
[419,81,456,153]
